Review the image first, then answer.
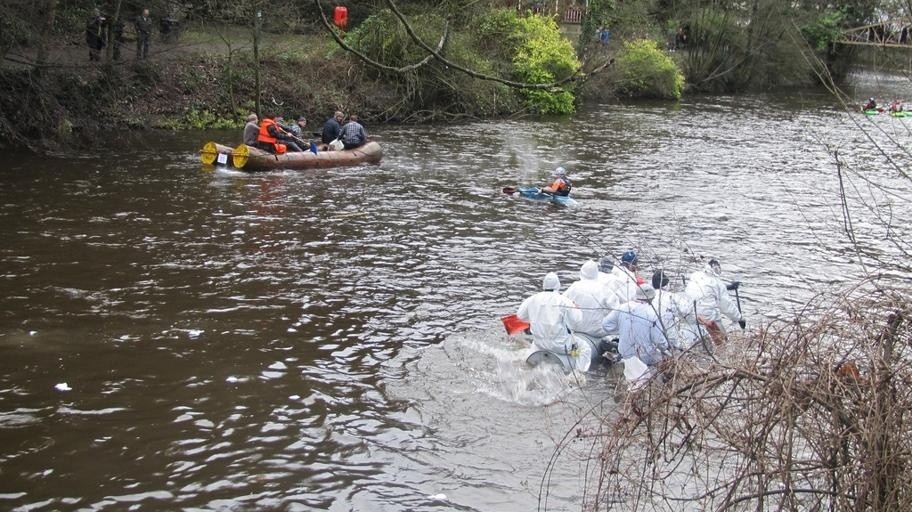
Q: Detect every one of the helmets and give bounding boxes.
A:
[552,167,566,177]
[542,250,722,300]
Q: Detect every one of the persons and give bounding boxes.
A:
[889,95,903,111]
[603,286,669,365]
[561,261,618,337]
[321,111,344,150]
[338,115,367,149]
[597,256,628,303]
[613,249,639,299]
[686,260,746,342]
[667,25,687,53]
[595,25,609,54]
[652,270,692,350]
[869,16,908,43]
[333,1,347,42]
[863,97,876,110]
[86,7,152,62]
[538,166,572,197]
[244,110,312,155]
[516,272,583,355]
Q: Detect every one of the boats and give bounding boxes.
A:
[524,284,750,410]
[200,136,389,174]
[504,186,578,214]
[904,110,912,117]
[887,105,905,117]
[856,100,881,117]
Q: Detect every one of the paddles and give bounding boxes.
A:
[503,188,538,193]
[278,127,316,154]
[501,315,529,335]
[699,317,724,345]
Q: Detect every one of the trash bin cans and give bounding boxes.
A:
[160,18,179,44]
